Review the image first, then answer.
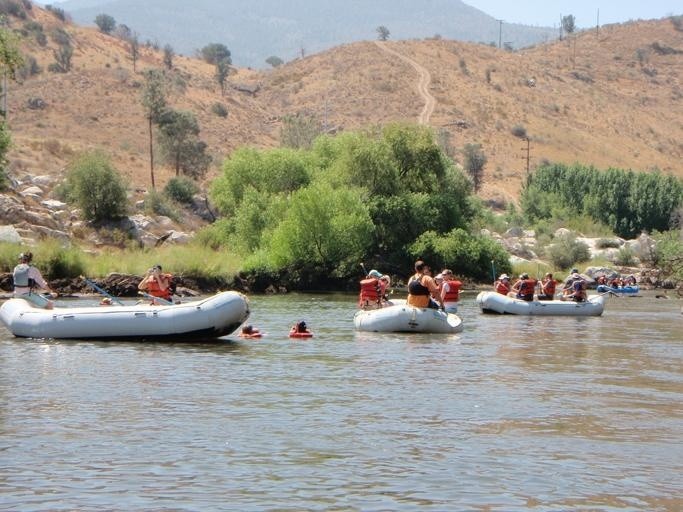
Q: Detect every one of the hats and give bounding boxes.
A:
[384,274,390,283]
[369,269,383,278]
[519,272,530,280]
[498,273,509,281]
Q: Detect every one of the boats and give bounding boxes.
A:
[595,284,639,293]
[474,290,606,317]
[0,287,255,340]
[349,298,466,335]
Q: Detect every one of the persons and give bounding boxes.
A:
[290,321,310,333]
[495,269,587,302]
[360,261,462,314]
[138,265,172,305]
[13,252,57,309]
[598,275,636,289]
[100,298,113,305]
[242,325,259,335]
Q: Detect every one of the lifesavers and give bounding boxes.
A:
[289,326,314,338]
[238,328,260,337]
